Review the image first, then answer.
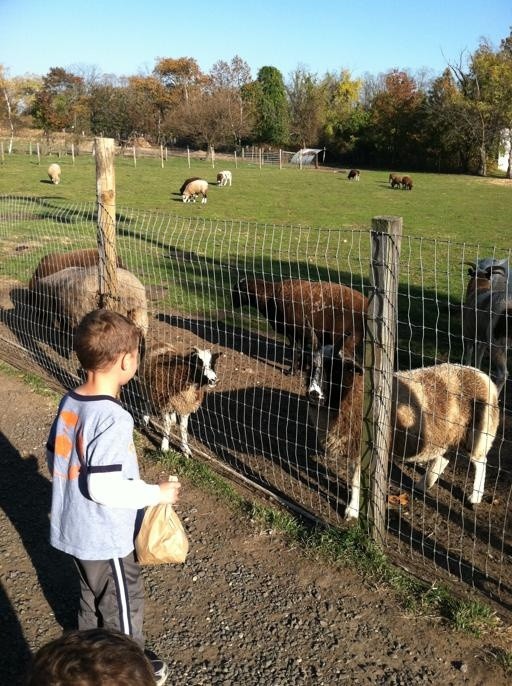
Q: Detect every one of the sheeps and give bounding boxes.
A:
[347,170,360,181]
[217,170,232,187]
[179,177,208,205]
[302,317,501,524]
[229,277,370,375]
[137,336,223,459]
[458,257,512,400]
[48,163,62,185]
[27,246,150,349]
[389,173,413,190]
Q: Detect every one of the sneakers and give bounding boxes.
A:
[149,659,169,686]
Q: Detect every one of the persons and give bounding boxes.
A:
[46,310,181,686]
[26,627,158,686]
